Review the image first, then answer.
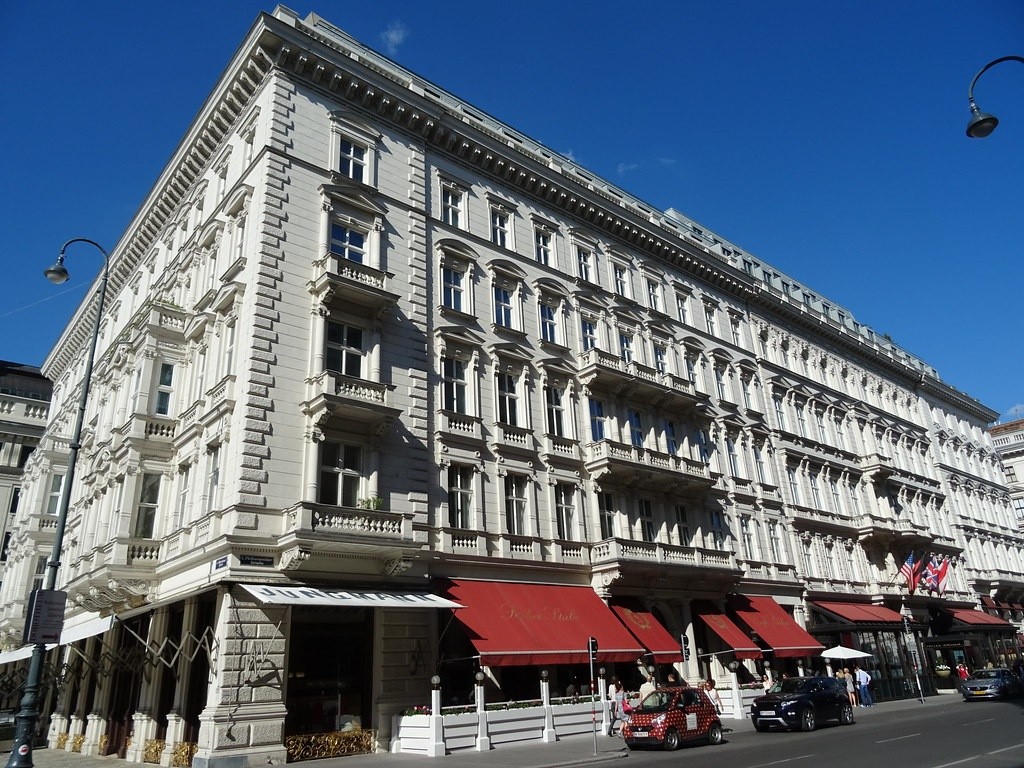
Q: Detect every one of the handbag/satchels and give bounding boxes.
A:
[622,700,633,713]
[716,704,721,715]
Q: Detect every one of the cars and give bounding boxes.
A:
[621,686,725,751]
[959,667,1018,700]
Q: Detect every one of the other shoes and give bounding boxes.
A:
[860,704,866,708]
[852,704,857,708]
[608,732,614,737]
[618,732,622,738]
[867,704,873,707]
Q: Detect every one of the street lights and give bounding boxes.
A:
[6,236,110,767]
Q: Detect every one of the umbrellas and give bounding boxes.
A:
[820,645,873,668]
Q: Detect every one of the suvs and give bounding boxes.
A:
[751,677,855,732]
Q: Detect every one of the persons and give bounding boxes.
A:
[639,676,657,705]
[705,679,724,712]
[956,653,1024,683]
[608,675,618,737]
[856,665,873,707]
[835,669,847,689]
[844,668,857,707]
[697,682,715,705]
[666,674,679,688]
[615,684,629,738]
[760,674,776,694]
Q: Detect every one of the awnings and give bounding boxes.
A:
[945,608,1010,624]
[728,595,825,657]
[692,605,763,659]
[609,601,684,663]
[815,600,904,622]
[0,585,214,664]
[239,582,466,609]
[442,578,647,666]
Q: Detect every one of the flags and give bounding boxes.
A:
[900,552,952,596]
